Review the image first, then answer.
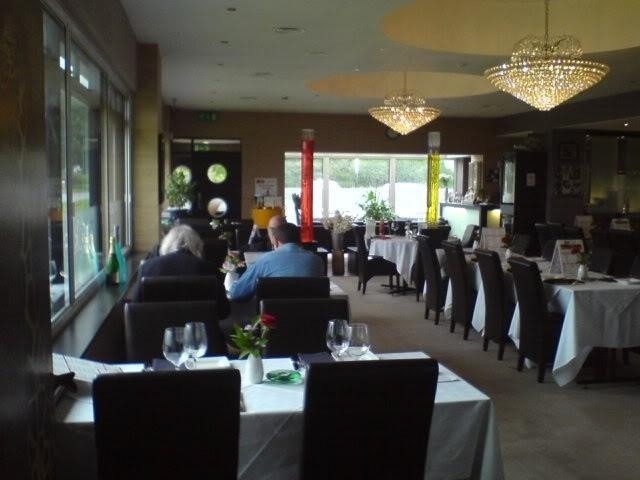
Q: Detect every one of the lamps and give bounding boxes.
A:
[484,2,610,112]
[367,72,442,137]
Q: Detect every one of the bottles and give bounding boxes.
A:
[114,225,127,283]
[105,237,120,286]
[248,225,264,251]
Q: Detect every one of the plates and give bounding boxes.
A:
[267,369,301,383]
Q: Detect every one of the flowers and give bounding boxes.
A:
[231,313,275,360]
[321,210,352,232]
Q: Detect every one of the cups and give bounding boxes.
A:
[347,322,370,360]
[160,325,191,373]
[325,318,351,362]
[181,321,209,372]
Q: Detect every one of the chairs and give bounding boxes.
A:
[133,274,217,325]
[92,368,241,480]
[300,358,439,480]
[440,239,479,340]
[474,249,516,360]
[507,256,563,382]
[450,224,479,248]
[402,225,451,302]
[256,275,331,320]
[236,228,268,250]
[203,238,227,266]
[124,301,222,364]
[259,295,350,359]
[415,234,449,325]
[352,224,400,294]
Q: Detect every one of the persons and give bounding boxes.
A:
[224,223,324,302]
[250,216,288,252]
[132,225,230,320]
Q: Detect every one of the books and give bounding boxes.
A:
[296,352,334,370]
[153,358,189,374]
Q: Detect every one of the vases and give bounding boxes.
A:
[379,220,385,239]
[246,353,264,385]
[331,233,344,250]
